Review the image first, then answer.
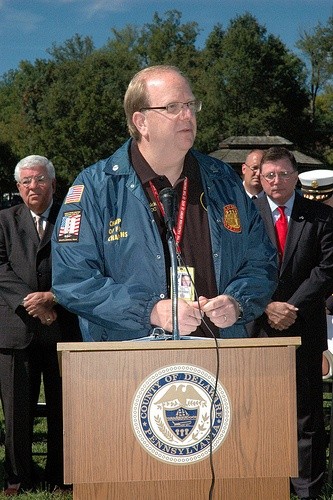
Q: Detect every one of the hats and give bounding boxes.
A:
[299,168,333,201]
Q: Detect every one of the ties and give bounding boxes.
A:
[34,215,45,241]
[274,206,289,263]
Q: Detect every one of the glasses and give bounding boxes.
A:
[260,170,297,184]
[244,163,261,173]
[20,175,52,188]
[141,99,202,118]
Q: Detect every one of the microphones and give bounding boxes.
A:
[159,187,177,240]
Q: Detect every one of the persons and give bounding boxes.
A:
[182,276,191,285]
[0,155,83,493]
[252,146,333,500]
[241,149,267,200]
[296,169,333,480]
[52,67,279,342]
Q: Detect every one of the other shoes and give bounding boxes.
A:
[10,472,70,492]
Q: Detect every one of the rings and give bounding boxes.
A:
[223,315,226,321]
[46,318,51,321]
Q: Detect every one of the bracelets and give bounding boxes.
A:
[50,290,57,302]
